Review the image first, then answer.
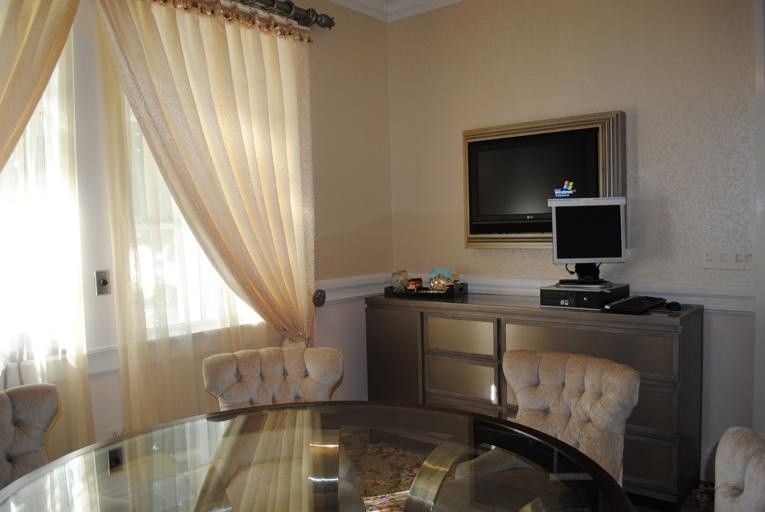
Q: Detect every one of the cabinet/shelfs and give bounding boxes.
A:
[364,293,704,505]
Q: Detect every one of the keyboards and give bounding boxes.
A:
[605,295,666,314]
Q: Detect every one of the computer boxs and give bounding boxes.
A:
[539,284,629,309]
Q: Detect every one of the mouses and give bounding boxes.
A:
[666,301,681,311]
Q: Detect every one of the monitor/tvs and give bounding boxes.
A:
[468,126,600,233]
[552,204,626,288]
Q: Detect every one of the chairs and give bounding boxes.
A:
[202,346,345,414]
[0,383,60,492]
[501,350,641,489]
[713,426,765,512]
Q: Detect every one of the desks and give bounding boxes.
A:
[0,400,641,512]
